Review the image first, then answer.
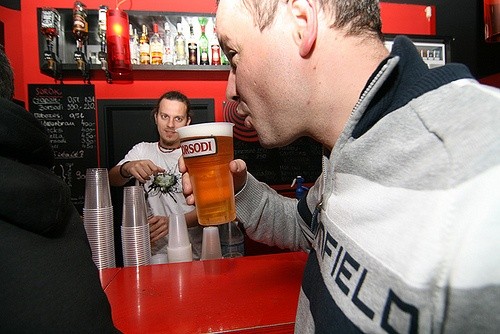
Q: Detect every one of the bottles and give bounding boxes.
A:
[72,1,89,52]
[96,5,108,52]
[295,175,304,201]
[129,18,230,65]
[40,7,61,52]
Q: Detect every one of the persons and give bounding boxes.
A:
[0,49,123,334]
[215,0,500,334]
[108,91,238,261]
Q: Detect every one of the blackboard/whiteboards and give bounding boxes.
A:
[27,83,101,205]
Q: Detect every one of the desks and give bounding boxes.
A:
[96,249,309,334]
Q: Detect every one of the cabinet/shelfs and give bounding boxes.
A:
[383,33,456,69]
[36,6,233,70]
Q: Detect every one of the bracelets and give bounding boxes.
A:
[119,161,133,178]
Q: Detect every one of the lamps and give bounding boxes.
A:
[105,8,133,75]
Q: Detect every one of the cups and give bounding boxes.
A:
[175,122,236,226]
[120,186,222,267]
[83,168,115,270]
